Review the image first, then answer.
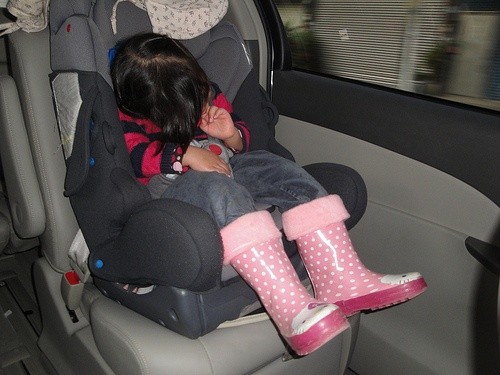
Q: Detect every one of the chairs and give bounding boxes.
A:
[0,1,368,375]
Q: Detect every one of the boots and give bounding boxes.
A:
[219,209,351,356]
[281,194,428,318]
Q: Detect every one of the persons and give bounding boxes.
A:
[110,32,428,356]
